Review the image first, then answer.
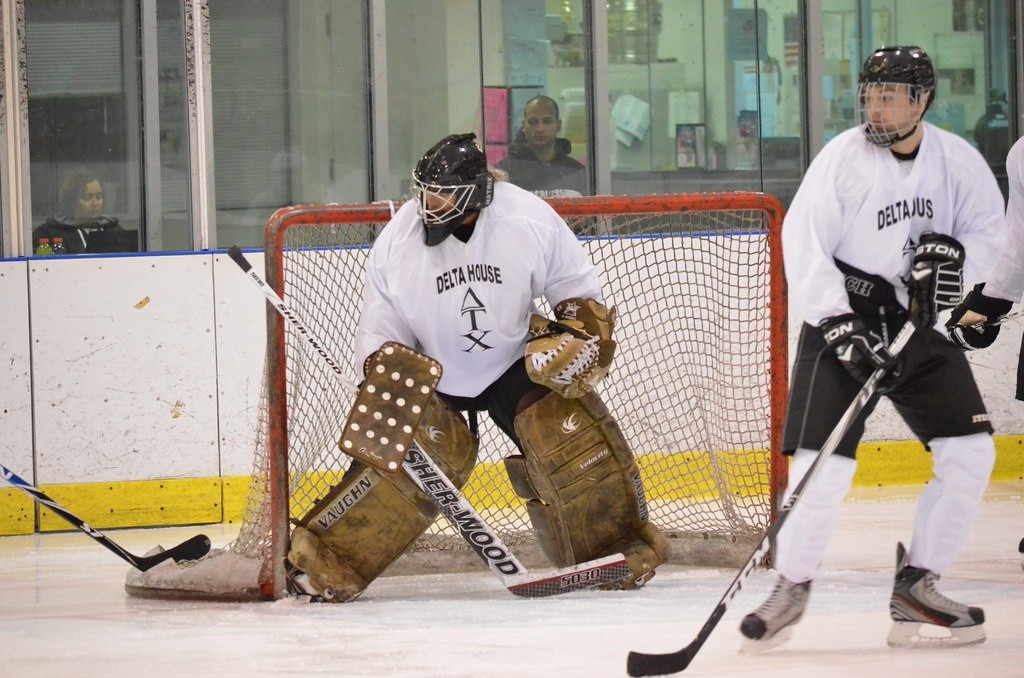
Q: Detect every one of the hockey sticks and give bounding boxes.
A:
[1,465,211,573]
[628,319,917,678]
[227,243,627,600]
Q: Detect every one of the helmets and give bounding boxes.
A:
[858,47,937,148]
[416,133,493,245]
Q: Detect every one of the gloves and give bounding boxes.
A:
[945,282,1013,351]
[819,313,903,395]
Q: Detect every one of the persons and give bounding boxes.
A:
[490,96,592,240]
[942,134,1024,555]
[280,131,675,603]
[738,43,1007,641]
[972,87,1010,206]
[32,168,131,256]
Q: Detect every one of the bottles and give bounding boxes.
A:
[36,239,52,255]
[52,238,66,255]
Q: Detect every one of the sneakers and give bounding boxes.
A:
[886,540,986,647]
[740,574,811,649]
